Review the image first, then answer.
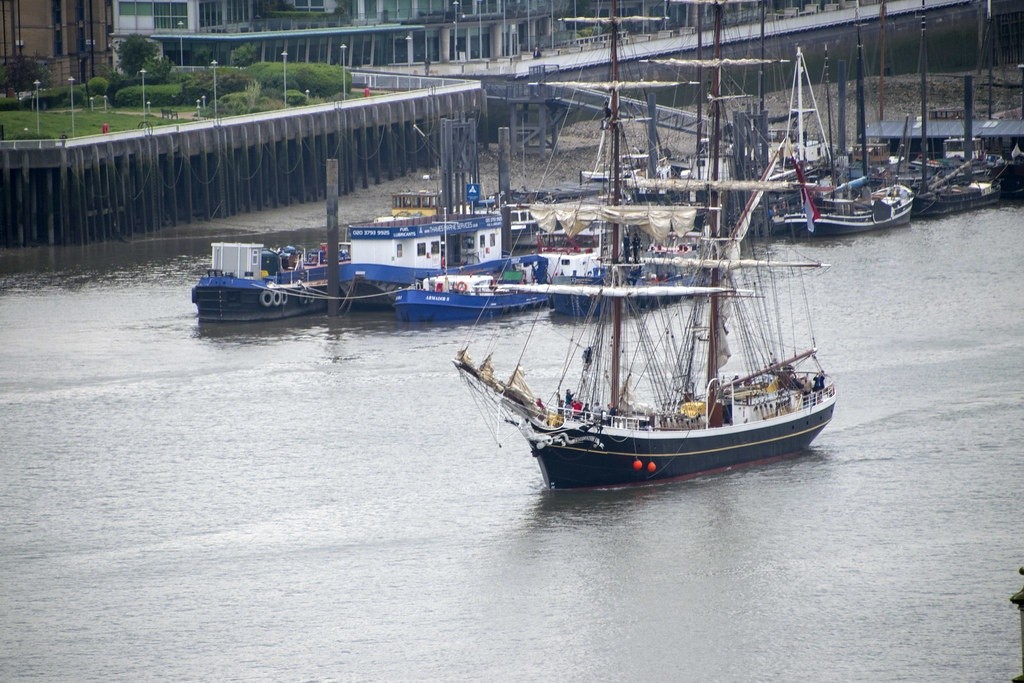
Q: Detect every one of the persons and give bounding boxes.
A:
[630,145,640,154]
[559,390,616,426]
[801,372,824,405]
[582,346,592,370]
[599,103,612,129]
[623,231,640,264]
[533,47,541,58]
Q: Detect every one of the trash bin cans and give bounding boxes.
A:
[364,88,370,97]
[102,123,109,134]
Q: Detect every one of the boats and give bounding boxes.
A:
[190,0,1024,327]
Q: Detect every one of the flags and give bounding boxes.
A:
[791,156,821,232]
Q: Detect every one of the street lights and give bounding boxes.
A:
[339,43,348,102]
[177,20,184,68]
[451,0,459,59]
[68,75,76,139]
[404,35,412,95]
[139,67,148,130]
[280,50,289,111]
[33,79,41,140]
[211,59,219,118]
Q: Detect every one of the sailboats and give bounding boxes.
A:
[449,2,838,494]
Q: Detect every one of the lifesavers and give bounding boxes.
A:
[441,256,445,267]
[259,292,274,306]
[272,291,281,305]
[281,291,288,304]
[458,282,467,292]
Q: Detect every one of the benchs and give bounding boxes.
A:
[161,108,178,120]
